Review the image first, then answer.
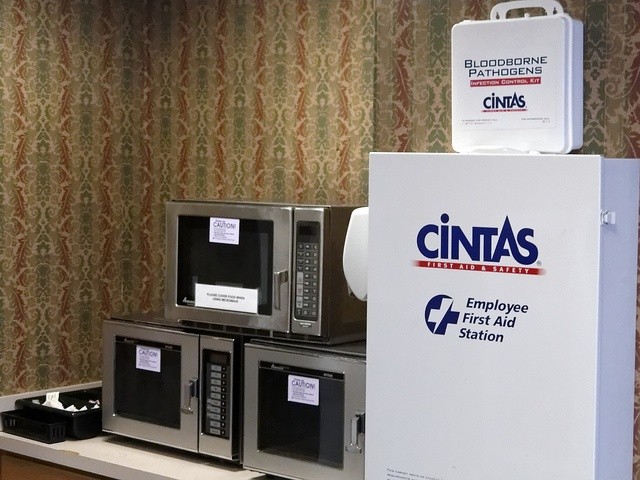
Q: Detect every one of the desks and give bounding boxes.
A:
[0,431,266,480]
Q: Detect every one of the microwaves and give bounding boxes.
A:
[165,198,366,347]
[242,338,366,480]
[102,314,238,463]
